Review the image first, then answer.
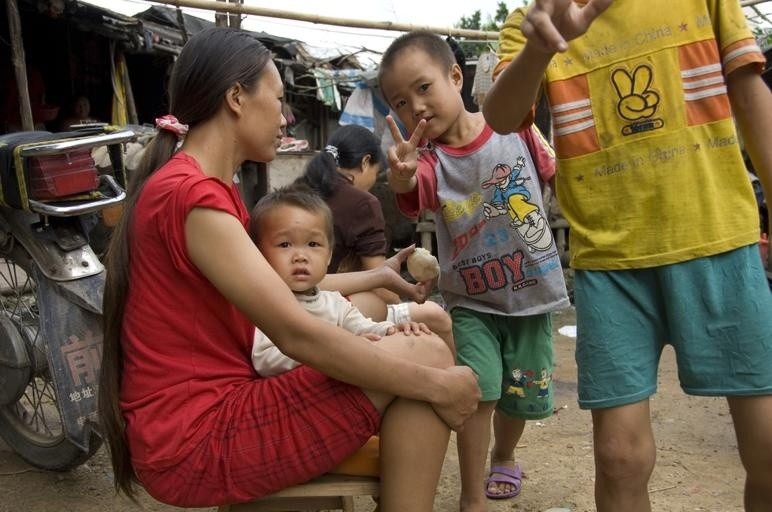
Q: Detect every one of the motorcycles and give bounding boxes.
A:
[0,119,137,471]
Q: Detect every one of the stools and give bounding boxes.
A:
[220,472,378,512]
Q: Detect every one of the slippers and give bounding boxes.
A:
[485,464,522,498]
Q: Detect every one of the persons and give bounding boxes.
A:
[277,120,405,305]
[376,29,573,503]
[91,24,486,510]
[474,1,772,512]
[249,183,437,380]
